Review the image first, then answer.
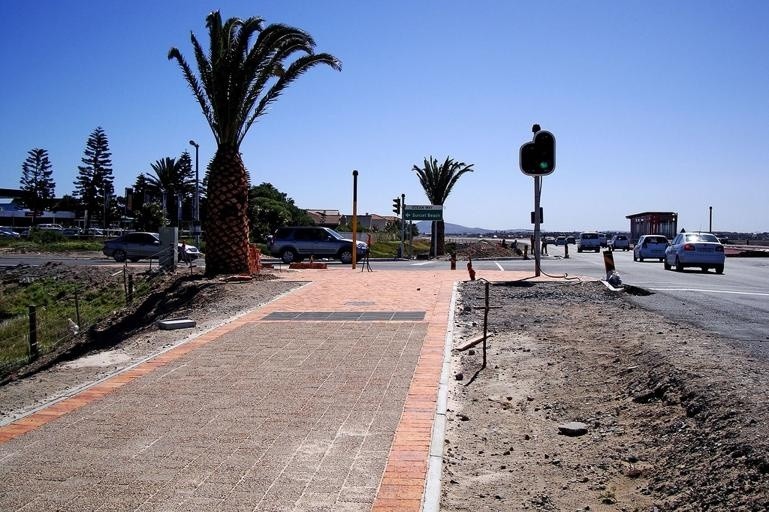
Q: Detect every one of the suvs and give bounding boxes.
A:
[269,225,368,265]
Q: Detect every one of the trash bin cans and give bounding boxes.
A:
[159,227,178,265]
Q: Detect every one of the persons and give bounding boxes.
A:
[531,235,535,254]
[502,238,507,248]
[541,236,548,254]
[511,240,518,248]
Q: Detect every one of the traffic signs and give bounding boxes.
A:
[403,205,442,220]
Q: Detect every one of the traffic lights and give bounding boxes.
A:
[519,128,556,176]
[392,198,400,214]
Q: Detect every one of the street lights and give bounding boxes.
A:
[189,140,200,231]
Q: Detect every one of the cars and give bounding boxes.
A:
[0,221,120,238]
[663,233,724,274]
[102,231,199,263]
[544,231,629,253]
[633,234,668,261]
